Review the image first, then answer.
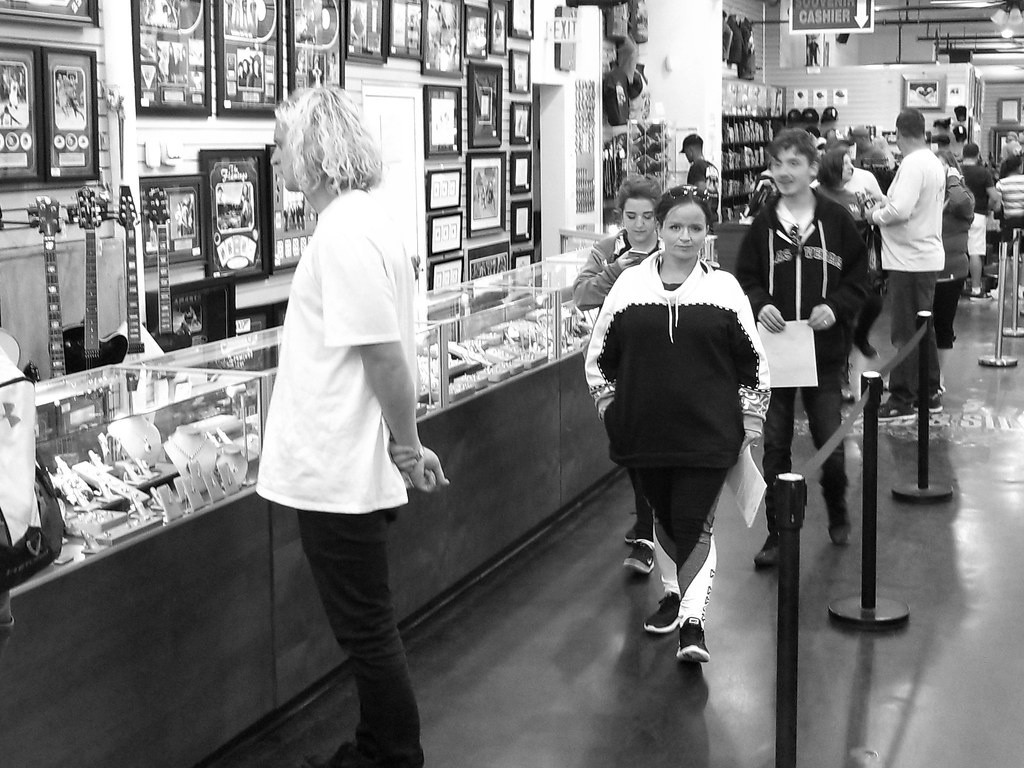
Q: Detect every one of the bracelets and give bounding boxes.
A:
[415,444,425,461]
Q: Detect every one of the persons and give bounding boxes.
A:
[586,185,773,665]
[55,71,85,120]
[678,134,721,230]
[574,171,671,310]
[225,0,259,38]
[935,130,1024,349]
[283,199,307,232]
[864,108,947,421]
[238,55,262,87]
[734,128,872,568]
[159,2,177,29]
[175,192,194,236]
[253,80,450,768]
[218,181,253,228]
[0,68,24,127]
[157,38,188,83]
[810,123,900,360]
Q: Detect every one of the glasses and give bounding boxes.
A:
[667,187,710,201]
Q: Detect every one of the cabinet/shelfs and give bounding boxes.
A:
[882,130,932,162]
[721,77,787,221]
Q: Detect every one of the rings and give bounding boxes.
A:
[823,320,828,326]
[753,444,758,447]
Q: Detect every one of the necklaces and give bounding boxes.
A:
[47,410,238,542]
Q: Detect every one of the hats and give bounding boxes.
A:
[680,134,703,153]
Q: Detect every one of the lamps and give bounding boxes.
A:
[990,1,1024,39]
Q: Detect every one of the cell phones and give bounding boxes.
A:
[627,250,648,265]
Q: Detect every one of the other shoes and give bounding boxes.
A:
[914,393,943,413]
[855,342,877,359]
[878,404,916,422]
[968,289,993,301]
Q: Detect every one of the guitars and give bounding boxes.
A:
[22,182,192,381]
[0,205,21,369]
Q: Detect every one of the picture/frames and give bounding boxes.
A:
[511,199,532,245]
[214,0,283,118]
[998,97,1022,124]
[0,0,100,27]
[139,144,317,285]
[285,0,535,99]
[430,257,464,290]
[510,101,531,145]
[428,211,463,257]
[990,126,1024,165]
[900,74,946,112]
[510,150,532,195]
[427,168,462,211]
[0,43,100,184]
[466,59,503,149]
[131,0,212,117]
[467,151,507,239]
[512,249,534,269]
[423,84,462,160]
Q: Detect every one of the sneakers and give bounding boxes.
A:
[826,502,851,546]
[643,592,681,634]
[676,618,711,662]
[754,534,781,565]
[622,542,655,572]
[623,520,638,542]
